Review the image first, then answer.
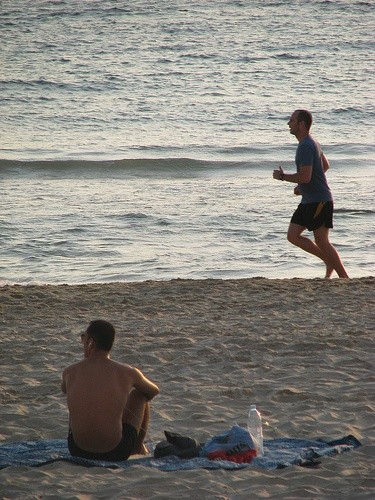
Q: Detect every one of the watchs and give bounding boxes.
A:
[279,174,284,180]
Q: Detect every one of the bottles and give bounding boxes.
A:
[248,404,265,456]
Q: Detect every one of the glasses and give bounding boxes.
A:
[80,333,89,342]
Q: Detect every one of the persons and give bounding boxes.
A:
[60,319,160,462]
[272,110,349,280]
